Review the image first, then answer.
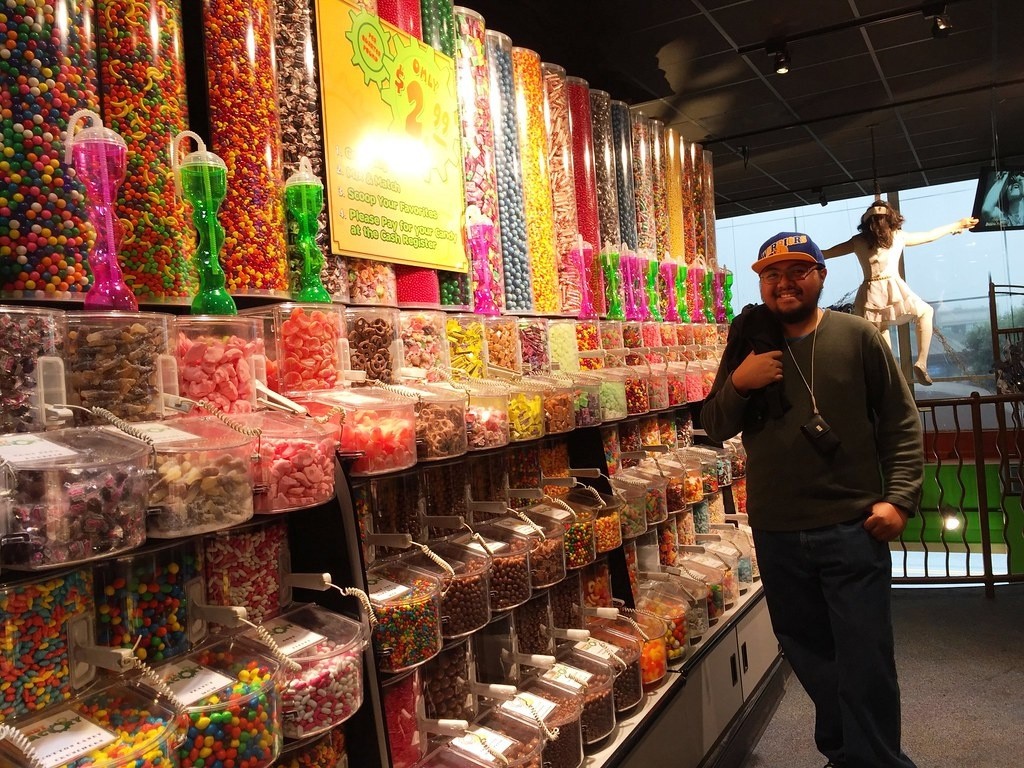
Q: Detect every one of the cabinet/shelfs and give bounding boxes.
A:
[681,585,784,761]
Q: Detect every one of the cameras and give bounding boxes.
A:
[800,413,842,456]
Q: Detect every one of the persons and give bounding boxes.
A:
[982,170,1024,226]
[821,200,979,387]
[703,228,927,768]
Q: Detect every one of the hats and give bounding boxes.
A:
[749,231,827,269]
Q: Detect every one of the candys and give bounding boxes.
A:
[0,0,759,767]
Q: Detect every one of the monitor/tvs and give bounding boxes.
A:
[969,166,1024,232]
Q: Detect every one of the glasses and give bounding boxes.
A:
[756,266,821,284]
